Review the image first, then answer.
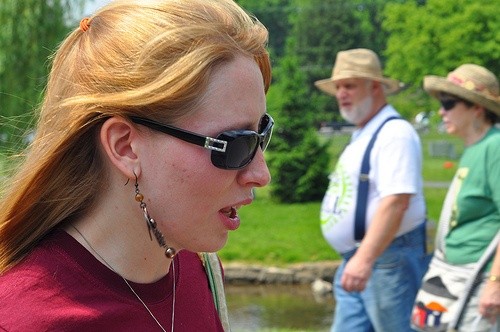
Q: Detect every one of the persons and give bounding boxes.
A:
[410,63,500,332]
[314,48,427,332]
[0,0,272,332]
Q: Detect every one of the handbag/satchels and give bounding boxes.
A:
[407,255,499,331]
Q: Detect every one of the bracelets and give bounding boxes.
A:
[483,275,499,281]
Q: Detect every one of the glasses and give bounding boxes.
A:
[439,98,474,111]
[124,113,276,171]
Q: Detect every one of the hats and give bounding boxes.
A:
[314,48,400,97]
[423,63,500,115]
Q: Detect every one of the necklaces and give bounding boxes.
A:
[67,220,177,332]
